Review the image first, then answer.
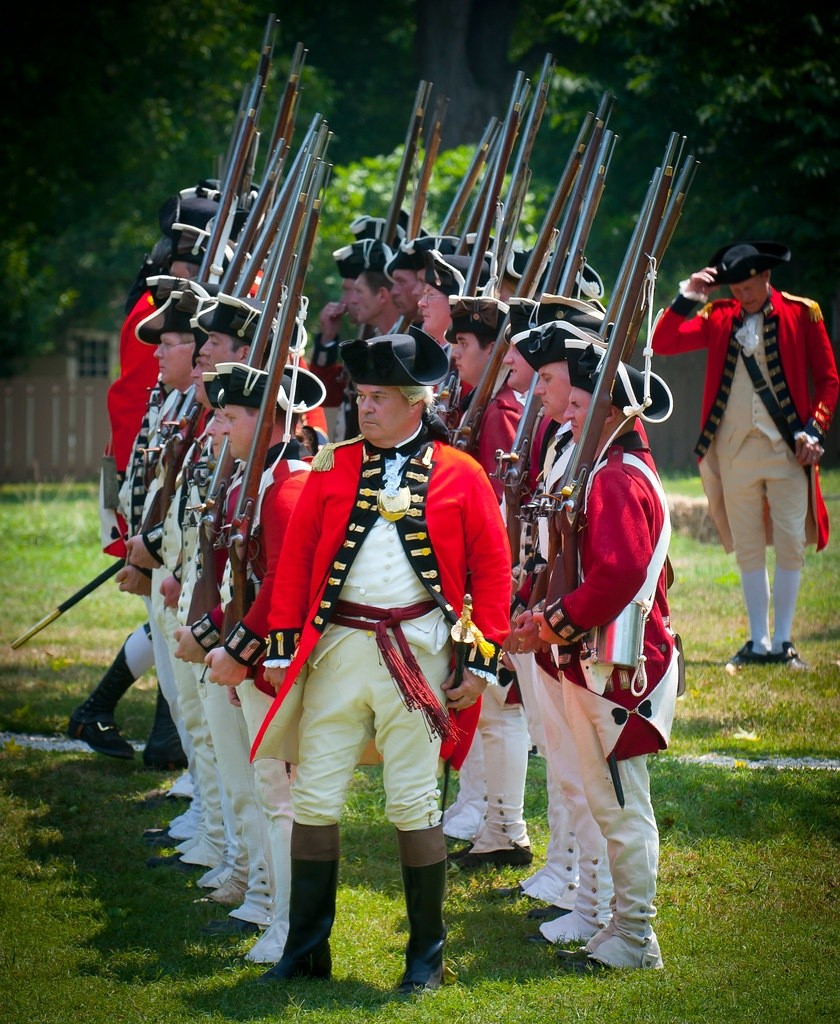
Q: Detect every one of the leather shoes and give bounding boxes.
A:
[557,948,594,960]
[561,959,610,975]
[527,934,552,944]
[528,904,572,919]
[492,886,539,905]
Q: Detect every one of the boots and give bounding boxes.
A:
[67,633,136,760]
[247,819,340,985]
[143,681,188,770]
[393,823,447,994]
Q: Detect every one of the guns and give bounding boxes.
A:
[382,48,700,608]
[141,12,341,639]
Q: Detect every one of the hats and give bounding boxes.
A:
[332,207,674,424]
[135,180,327,413]
[705,242,792,287]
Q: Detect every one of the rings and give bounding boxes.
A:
[517,648,525,652]
[469,699,477,705]
[812,456,818,459]
[533,648,539,653]
[518,636,527,642]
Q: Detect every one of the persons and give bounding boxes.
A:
[66,180,681,972]
[260,324,514,995]
[647,240,839,670]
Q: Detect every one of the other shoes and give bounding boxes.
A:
[716,641,771,665]
[448,840,534,873]
[768,642,809,670]
[141,774,260,935]
